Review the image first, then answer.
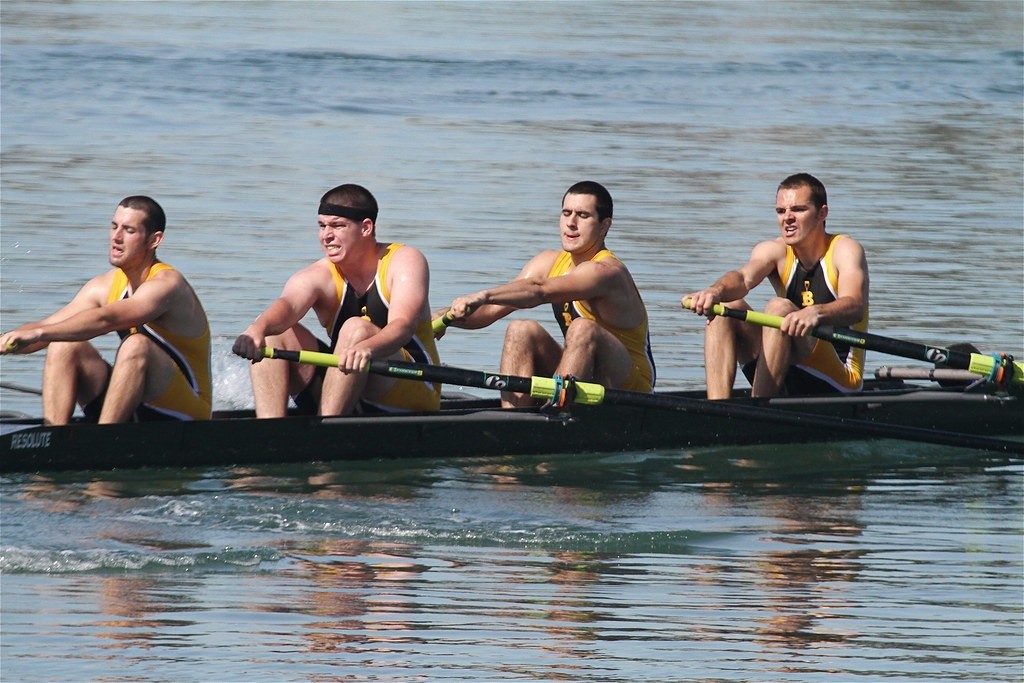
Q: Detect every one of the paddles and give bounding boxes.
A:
[431,306,472,333]
[680,297,1023,384]
[231,343,1023,455]
[0,341,20,355]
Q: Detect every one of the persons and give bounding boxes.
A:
[679,173,870,400]
[433,182,656,408]
[232,184,442,419]
[0,195,213,425]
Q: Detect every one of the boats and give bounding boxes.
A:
[0,344,1024,474]
